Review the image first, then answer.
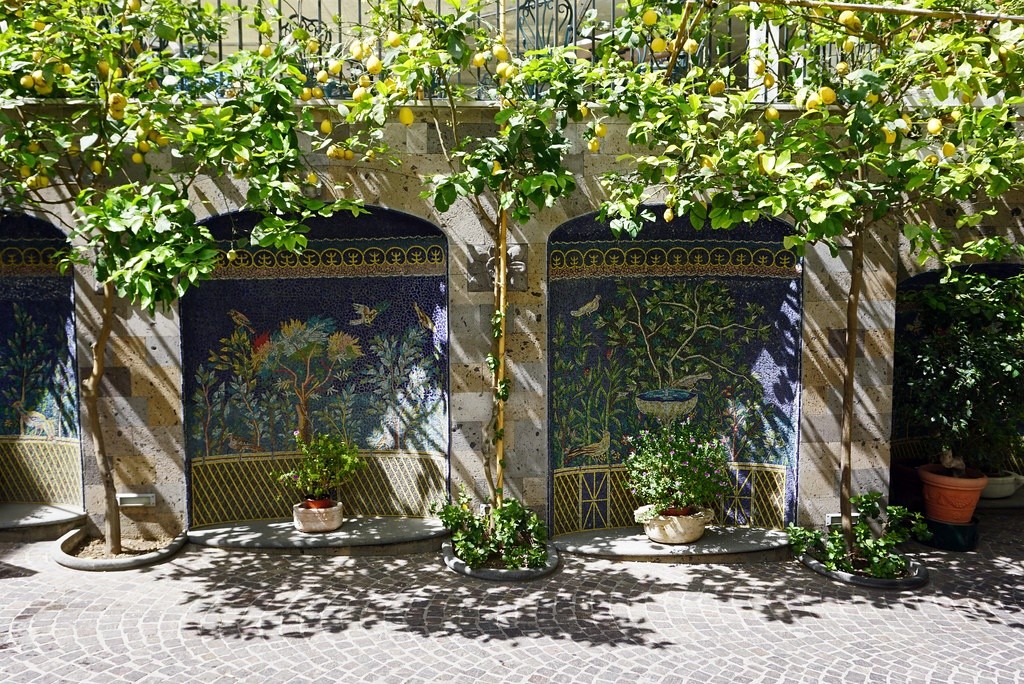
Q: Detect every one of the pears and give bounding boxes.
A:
[20,0,1011,223]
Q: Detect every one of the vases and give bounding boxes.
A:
[304,494,332,509]
[921,516,980,552]
[659,506,693,516]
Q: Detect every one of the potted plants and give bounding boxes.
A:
[904,320,1024,522]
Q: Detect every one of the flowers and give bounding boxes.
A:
[620,412,739,527]
[267,428,375,502]
